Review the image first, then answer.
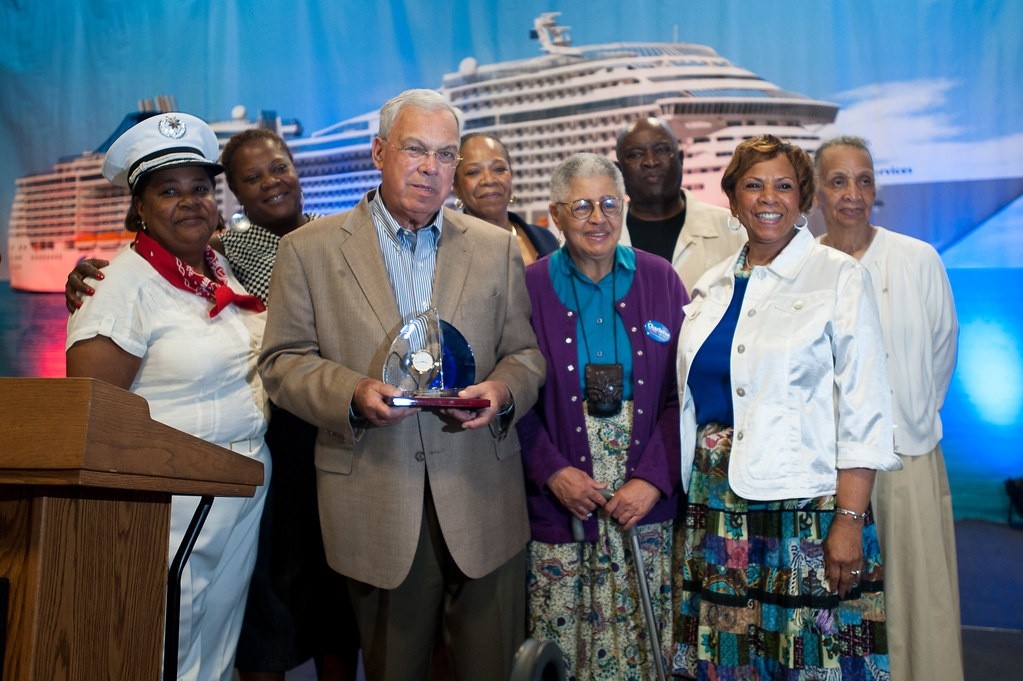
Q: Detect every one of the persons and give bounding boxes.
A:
[257,89,546,577]
[515,153,691,681]
[65,127,365,681]
[616,117,750,292]
[671,134,904,681]
[814,139,968,681]
[64,112,271,681]
[452,133,561,265]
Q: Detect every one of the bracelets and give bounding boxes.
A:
[837,508,869,519]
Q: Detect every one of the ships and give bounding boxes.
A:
[6,11,1023,298]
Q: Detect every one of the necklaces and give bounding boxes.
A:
[512,224,517,235]
[734,241,753,278]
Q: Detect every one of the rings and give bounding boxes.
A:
[851,570,859,574]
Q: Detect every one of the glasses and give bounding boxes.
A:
[556,198,624,219]
[384,138,464,169]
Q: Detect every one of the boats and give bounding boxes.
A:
[73,232,94,249]
[96,231,120,250]
[118,228,138,248]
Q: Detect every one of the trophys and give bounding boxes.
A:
[382,306,491,409]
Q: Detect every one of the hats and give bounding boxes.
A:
[99,112,225,194]
